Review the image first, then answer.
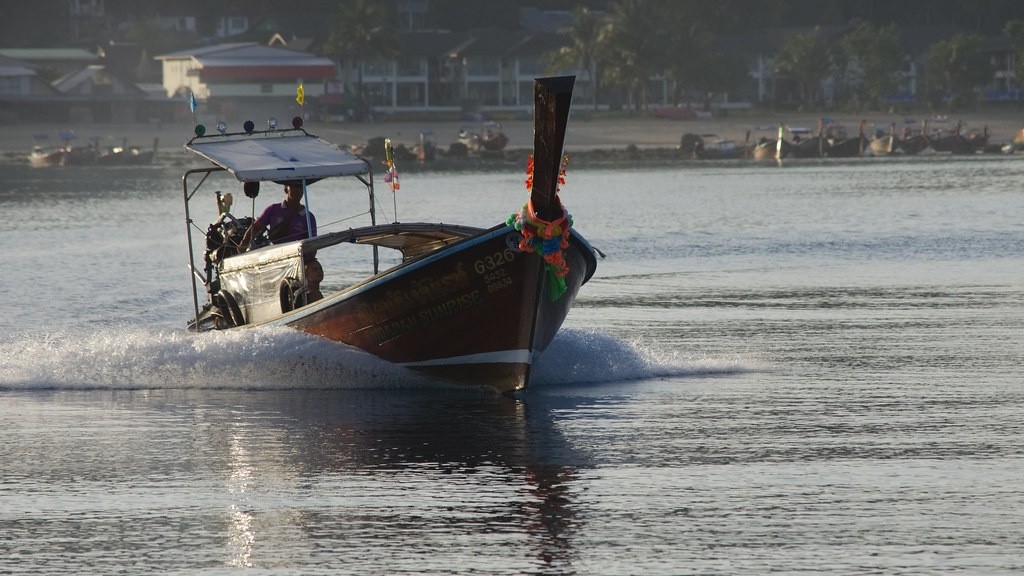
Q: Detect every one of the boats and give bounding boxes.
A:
[334,119,510,165]
[681,116,1023,160]
[184,76,600,397]
[0,130,159,170]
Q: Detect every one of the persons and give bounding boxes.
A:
[237,184,317,254]
[292,260,324,309]
